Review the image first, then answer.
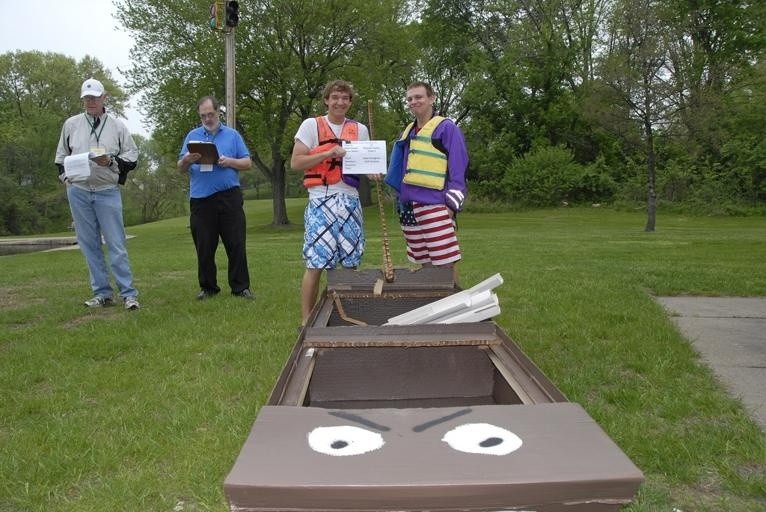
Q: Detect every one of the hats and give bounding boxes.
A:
[80,78,105,99]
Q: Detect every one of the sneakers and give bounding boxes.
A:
[123,296,140,310]
[233,289,256,301]
[198,289,216,300]
[84,293,117,308]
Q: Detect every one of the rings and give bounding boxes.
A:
[104,161,105,163]
[219,161,221,165]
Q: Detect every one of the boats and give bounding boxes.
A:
[221,258,647,512]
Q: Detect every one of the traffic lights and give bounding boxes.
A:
[223,0,239,29]
[208,0,225,31]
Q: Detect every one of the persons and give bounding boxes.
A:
[291,80,382,327]
[54,78,140,311]
[383,81,469,285]
[177,96,256,301]
